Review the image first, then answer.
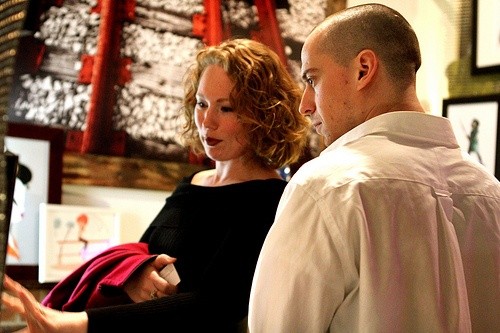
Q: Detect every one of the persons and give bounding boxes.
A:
[0,38,311,333]
[246,4,500,333]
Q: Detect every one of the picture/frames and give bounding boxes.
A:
[4,121,67,274]
[472,0,500,75]
[442,93,500,184]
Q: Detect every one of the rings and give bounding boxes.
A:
[150,289,158,298]
[38,303,44,315]
